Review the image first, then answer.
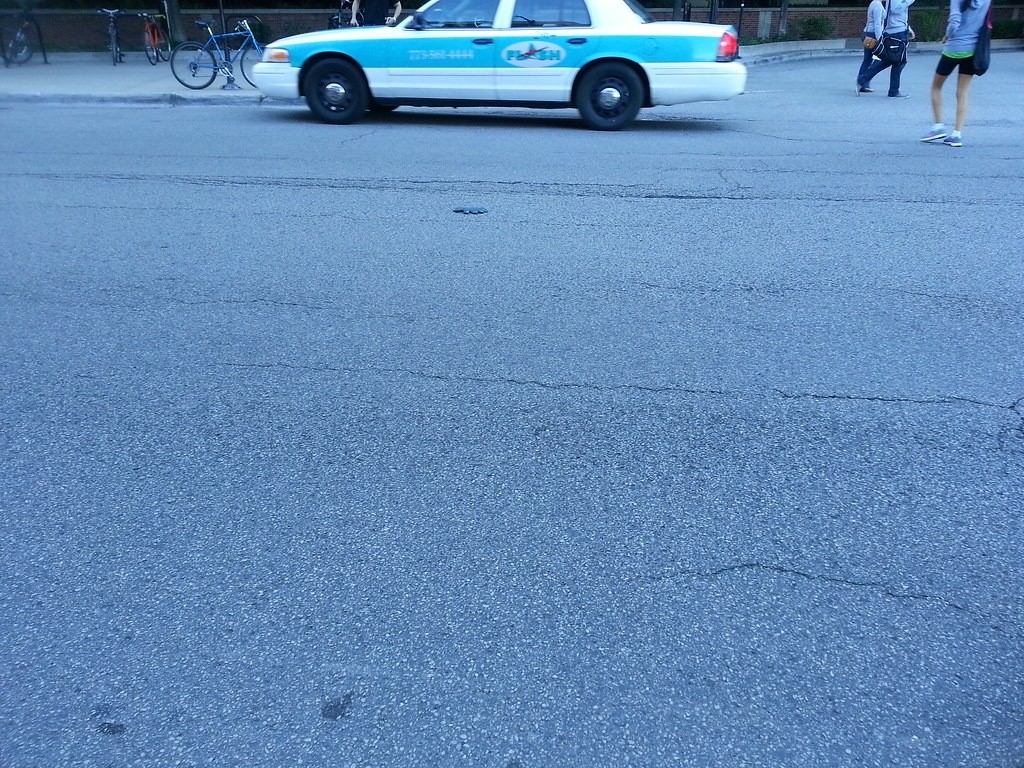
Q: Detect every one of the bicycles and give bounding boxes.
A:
[169,14,270,90]
[0,12,38,68]
[136,12,171,66]
[326,0,366,29]
[97,8,127,66]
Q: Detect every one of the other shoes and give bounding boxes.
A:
[859,87,873,92]
[888,93,909,99]
[856,76,862,95]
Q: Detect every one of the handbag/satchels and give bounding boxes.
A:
[863,36,876,50]
[872,32,909,66]
[973,6,993,76]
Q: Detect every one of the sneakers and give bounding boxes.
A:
[944,135,963,147]
[919,127,948,141]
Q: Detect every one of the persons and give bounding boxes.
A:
[855,0,917,99]
[920,0,990,147]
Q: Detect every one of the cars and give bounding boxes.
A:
[250,0,747,135]
